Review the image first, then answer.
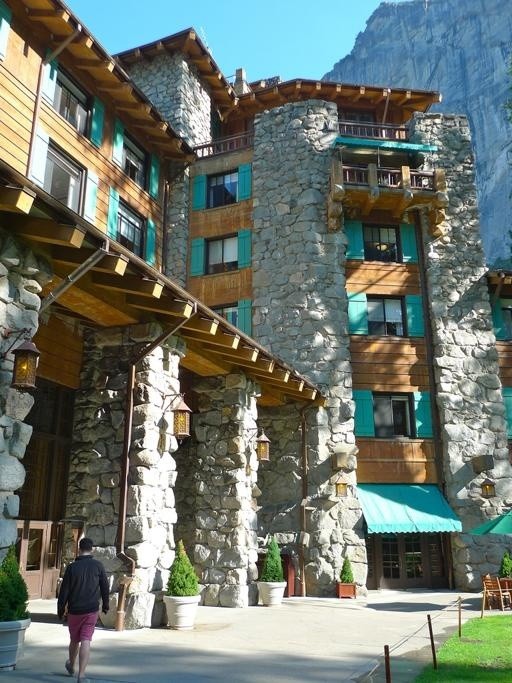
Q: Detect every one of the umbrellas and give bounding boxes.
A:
[470,508,512,535]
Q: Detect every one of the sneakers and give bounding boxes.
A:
[78,678,90,683]
[66,660,75,676]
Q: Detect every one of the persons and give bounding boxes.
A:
[57,538,111,681]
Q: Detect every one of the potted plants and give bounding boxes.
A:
[256,535,288,605]
[336,554,355,598]
[163,539,202,631]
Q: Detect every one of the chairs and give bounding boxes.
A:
[480,573,512,611]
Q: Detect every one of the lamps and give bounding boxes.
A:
[480,469,496,498]
[3,327,41,391]
[335,469,348,497]
[247,427,271,462]
[163,392,192,438]
